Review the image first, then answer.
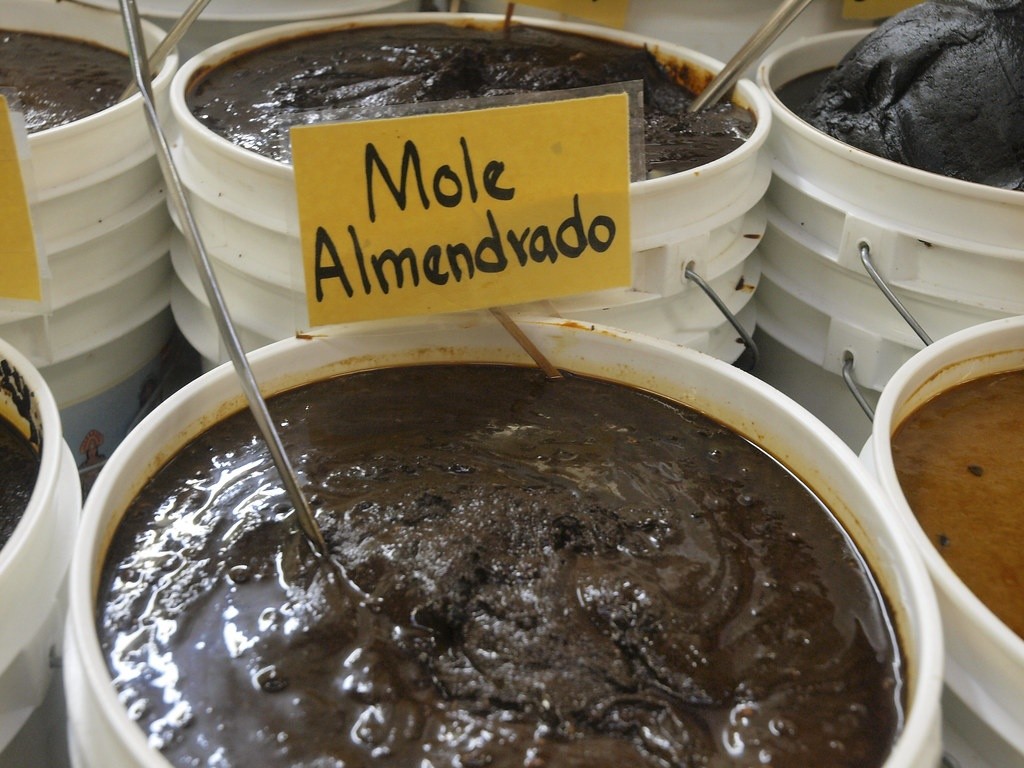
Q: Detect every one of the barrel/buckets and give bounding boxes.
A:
[0,0,1024,768]
